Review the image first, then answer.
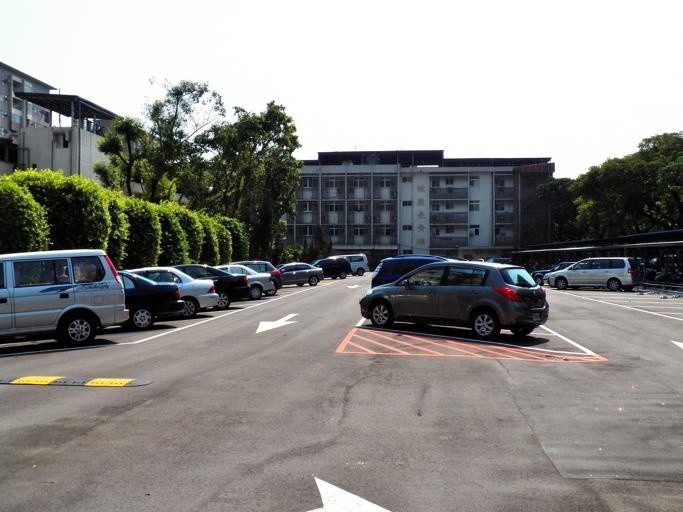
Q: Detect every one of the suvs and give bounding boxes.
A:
[359,260,550,341]
[369,254,463,290]
[530,261,588,290]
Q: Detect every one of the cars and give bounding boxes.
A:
[115,271,185,330]
[154,263,250,310]
[211,253,369,301]
[122,267,219,319]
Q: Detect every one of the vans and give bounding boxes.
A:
[0,248,129,347]
[547,255,644,292]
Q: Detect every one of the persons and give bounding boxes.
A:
[532,261,541,270]
